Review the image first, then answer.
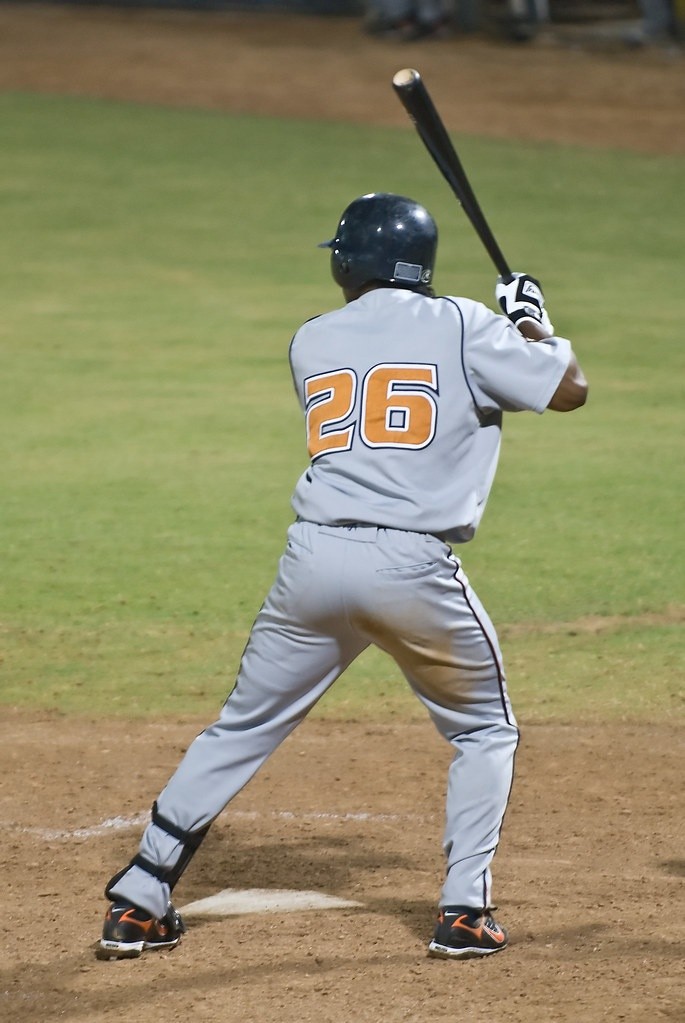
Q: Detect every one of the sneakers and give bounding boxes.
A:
[428,906,508,959]
[99,902,184,956]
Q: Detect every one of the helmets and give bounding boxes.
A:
[318,192,438,285]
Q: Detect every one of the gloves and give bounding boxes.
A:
[525,308,555,341]
[494,272,544,327]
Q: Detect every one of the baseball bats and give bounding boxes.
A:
[389,65,516,287]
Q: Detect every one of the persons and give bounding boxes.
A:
[96,194,587,958]
